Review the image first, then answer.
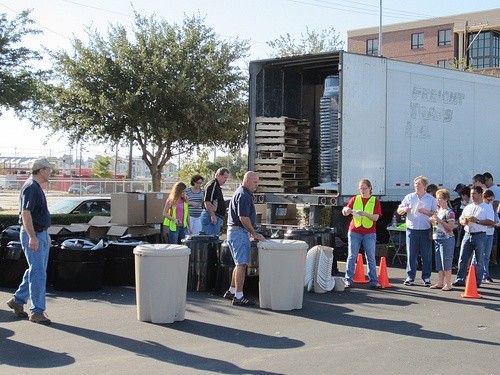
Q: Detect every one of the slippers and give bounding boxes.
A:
[442,286,453,291]
[429,285,443,289]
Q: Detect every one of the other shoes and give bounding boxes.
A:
[452,281,461,286]
[482,277,493,283]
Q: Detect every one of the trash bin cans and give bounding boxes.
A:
[256,239,309,311]
[182,231,219,292]
[1,225,139,292]
[133,242,193,325]
[219,225,339,297]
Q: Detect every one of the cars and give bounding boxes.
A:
[48,196,111,214]
[84,185,103,194]
[68,184,87,194]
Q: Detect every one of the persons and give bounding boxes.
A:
[6,157,55,325]
[185,175,204,234]
[201,166,230,236]
[396,176,439,287]
[428,189,455,290]
[452,187,496,288]
[163,182,193,245]
[224,171,267,307]
[343,179,382,288]
[427,173,500,284]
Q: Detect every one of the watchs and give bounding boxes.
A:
[476,220,480,224]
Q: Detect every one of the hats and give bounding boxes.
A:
[454,183,466,192]
[32,158,55,171]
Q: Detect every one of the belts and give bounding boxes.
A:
[470,231,486,235]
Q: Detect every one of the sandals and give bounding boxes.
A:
[344,284,353,288]
[371,283,385,289]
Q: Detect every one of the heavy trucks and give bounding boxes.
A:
[247,50,500,245]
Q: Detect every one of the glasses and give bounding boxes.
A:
[196,182,202,185]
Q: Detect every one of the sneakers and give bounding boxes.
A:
[30,312,50,325]
[422,278,431,287]
[403,277,414,285]
[7,298,28,317]
[224,290,235,301]
[233,296,255,306]
